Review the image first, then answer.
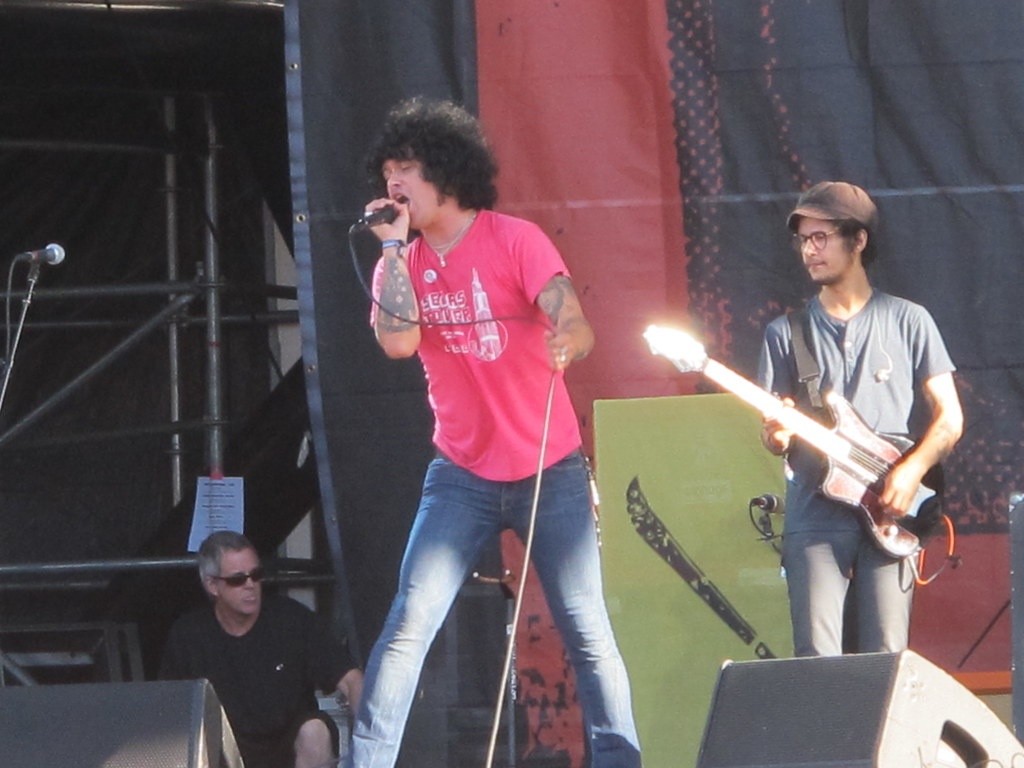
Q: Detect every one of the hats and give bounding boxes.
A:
[786,181,879,234]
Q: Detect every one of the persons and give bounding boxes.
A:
[761,181,965,657]
[159,531,363,768]
[344,100,641,768]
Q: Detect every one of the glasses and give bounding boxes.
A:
[212,567,266,587]
[792,226,846,251]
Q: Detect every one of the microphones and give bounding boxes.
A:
[17,243,65,266]
[754,494,786,515]
[353,204,398,233]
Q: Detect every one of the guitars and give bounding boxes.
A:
[641,319,940,559]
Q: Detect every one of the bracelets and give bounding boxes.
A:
[382,239,407,257]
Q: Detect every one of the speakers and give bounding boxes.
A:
[696,650,1024,768]
[0,678,244,768]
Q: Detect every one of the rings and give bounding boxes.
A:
[559,346,567,362]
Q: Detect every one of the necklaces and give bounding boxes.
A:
[429,214,476,267]
